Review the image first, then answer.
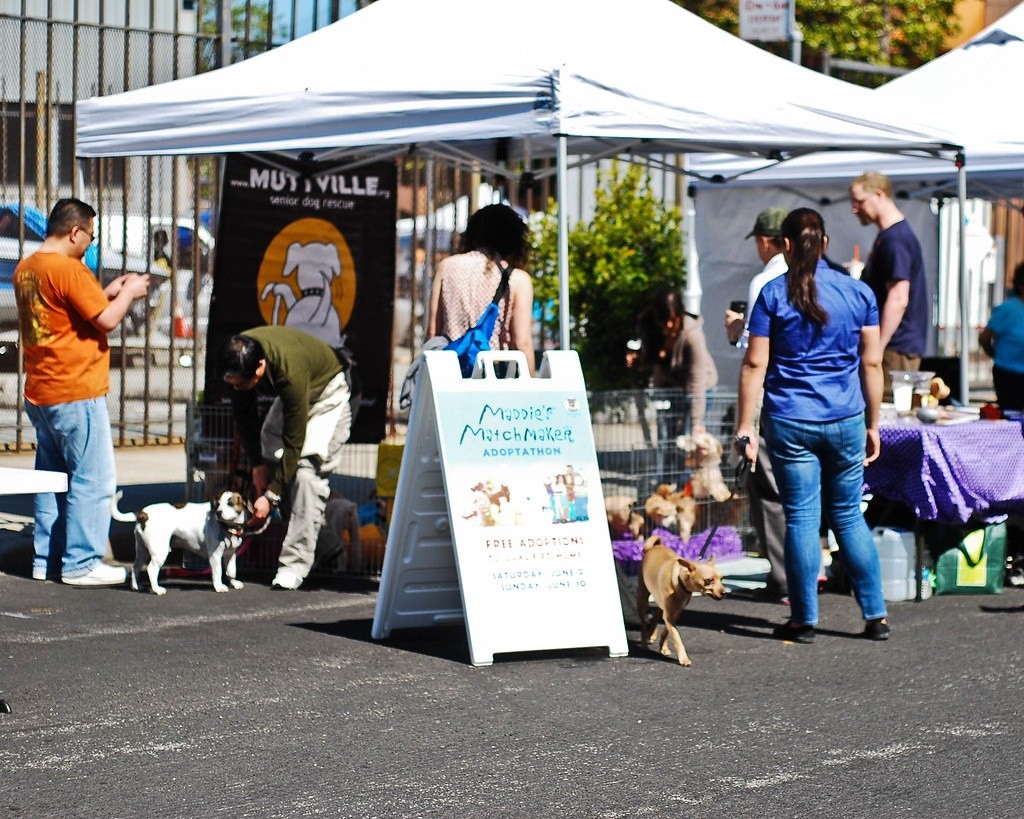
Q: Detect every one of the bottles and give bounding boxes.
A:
[730,301,747,346]
[918,524,932,598]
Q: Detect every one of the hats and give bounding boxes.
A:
[745,207,790,240]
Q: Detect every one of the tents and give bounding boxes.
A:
[72,0,970,408]
[690,0,1024,407]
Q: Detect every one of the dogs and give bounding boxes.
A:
[111,490,255,596]
[637,535,727,667]
[605,432,738,543]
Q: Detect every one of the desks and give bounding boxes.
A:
[862,407,1024,602]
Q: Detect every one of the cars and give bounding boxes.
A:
[0,199,169,337]
[395,260,427,348]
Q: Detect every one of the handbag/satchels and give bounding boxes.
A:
[934,513,1006,595]
[398,304,500,411]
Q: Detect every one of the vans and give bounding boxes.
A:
[94,213,215,340]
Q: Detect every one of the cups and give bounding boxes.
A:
[915,372,935,408]
[888,370,914,412]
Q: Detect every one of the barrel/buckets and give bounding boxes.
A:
[852,527,917,603]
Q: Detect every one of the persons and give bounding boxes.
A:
[846,170,928,404]
[422,201,535,381]
[724,208,790,603]
[734,208,891,640]
[976,261,1024,407]
[216,325,354,589]
[12,198,152,586]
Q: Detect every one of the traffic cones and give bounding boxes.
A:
[172,298,192,338]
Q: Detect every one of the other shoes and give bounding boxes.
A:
[272,567,304,591]
[773,620,817,643]
[758,588,790,607]
[864,617,891,640]
[33,560,128,585]
